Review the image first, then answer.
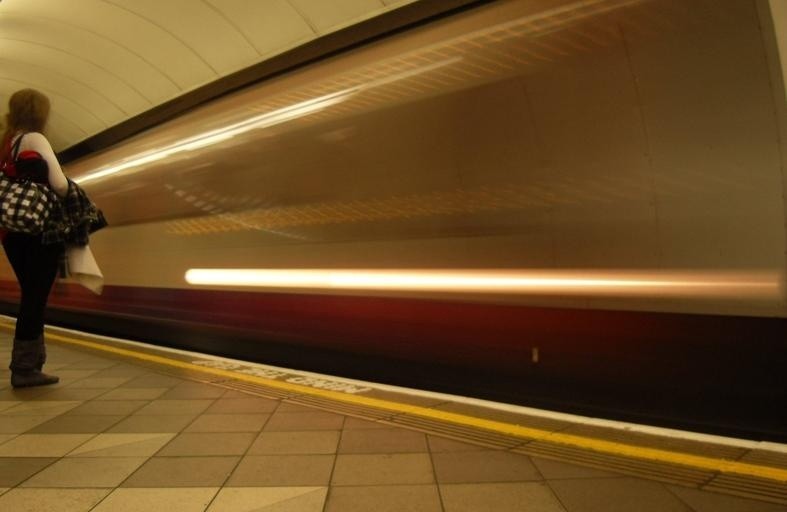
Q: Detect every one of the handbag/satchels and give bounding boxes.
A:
[0,172,60,236]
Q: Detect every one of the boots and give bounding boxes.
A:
[10,335,59,386]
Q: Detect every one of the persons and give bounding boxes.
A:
[0,89,74,388]
